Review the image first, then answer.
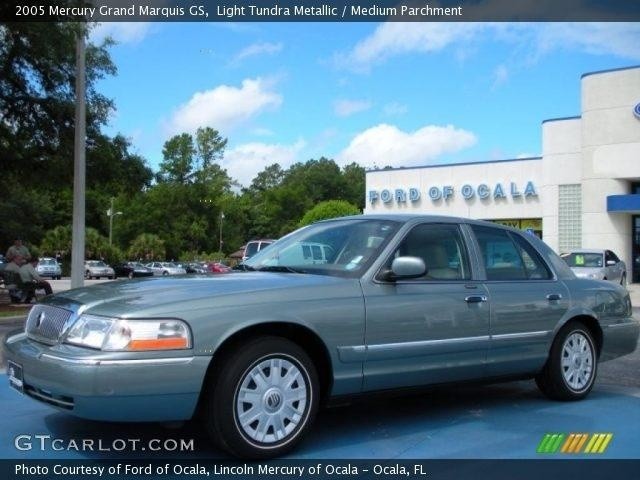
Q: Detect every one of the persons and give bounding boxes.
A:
[20,257,53,296]
[0,255,33,304]
[5,237,31,268]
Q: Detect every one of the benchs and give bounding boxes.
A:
[0,270,39,304]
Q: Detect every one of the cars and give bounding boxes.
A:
[0,255,234,278]
[6,212,640,462]
[554,249,630,287]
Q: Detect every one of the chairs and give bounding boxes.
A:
[417,245,460,281]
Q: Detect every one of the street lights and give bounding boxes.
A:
[108,199,122,244]
[219,212,227,254]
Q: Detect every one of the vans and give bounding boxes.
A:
[240,238,336,269]
